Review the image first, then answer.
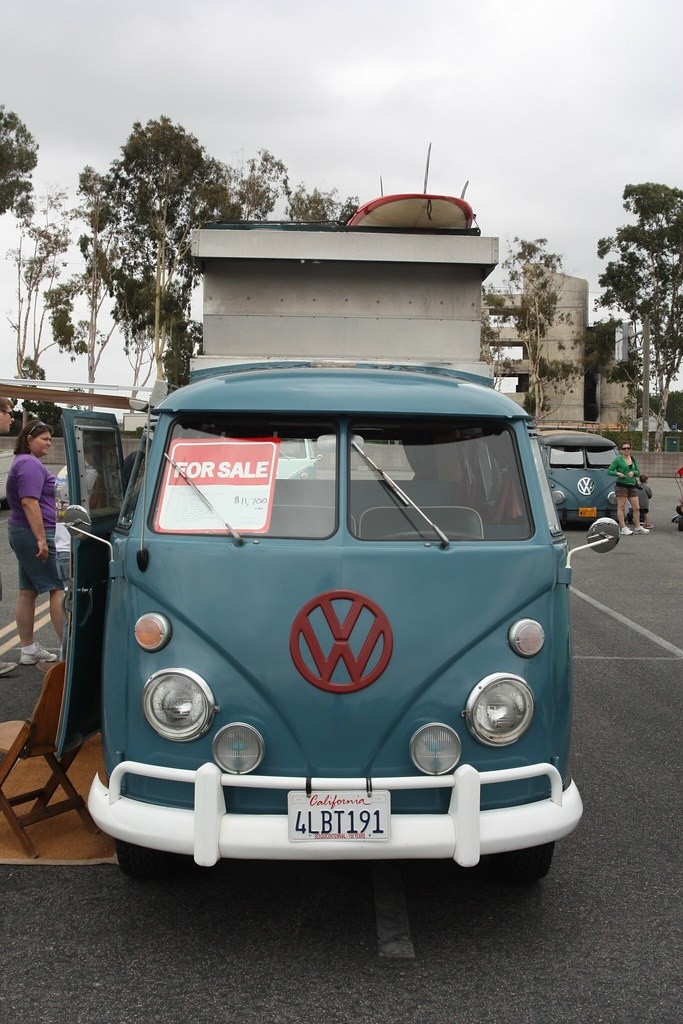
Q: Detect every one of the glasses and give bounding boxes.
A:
[621,447,631,451]
[28,422,46,435]
[0,409,14,418]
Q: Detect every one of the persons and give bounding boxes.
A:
[638,473,653,528]
[608,440,649,535]
[6,420,138,672]
[0,397,18,673]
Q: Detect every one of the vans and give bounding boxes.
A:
[59,358,623,890]
[536,429,634,529]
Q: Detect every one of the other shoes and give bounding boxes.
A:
[644,524,653,529]
[0,661,18,674]
[640,521,645,525]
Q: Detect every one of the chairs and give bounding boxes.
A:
[0,662,99,858]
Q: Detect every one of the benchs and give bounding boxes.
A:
[274,478,472,519]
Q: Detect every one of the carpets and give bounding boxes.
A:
[0,731,120,866]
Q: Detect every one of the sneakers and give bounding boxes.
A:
[634,526,650,535]
[19,643,58,665]
[619,527,633,535]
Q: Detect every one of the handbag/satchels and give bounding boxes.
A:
[630,455,643,489]
